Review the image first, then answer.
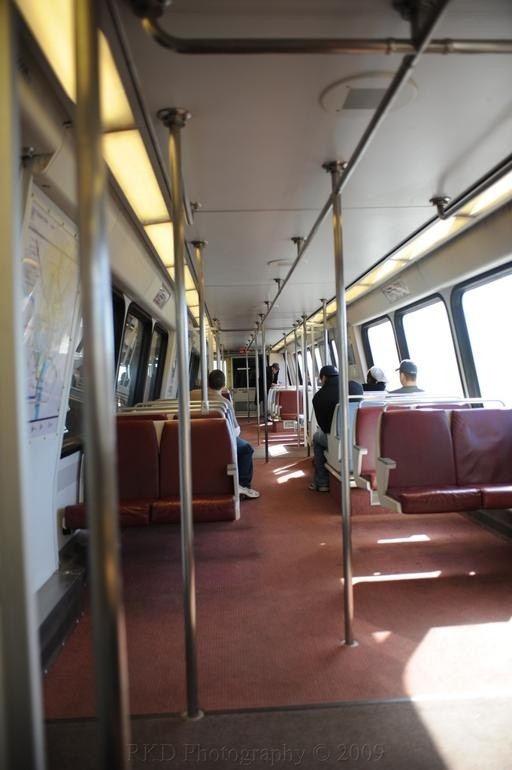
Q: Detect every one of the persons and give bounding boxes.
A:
[361,365,388,390]
[389,359,423,393]
[309,366,362,493]
[254,363,281,422]
[188,369,260,499]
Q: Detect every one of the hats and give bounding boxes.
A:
[320,365,339,376]
[395,360,418,375]
[370,366,385,382]
[272,363,281,371]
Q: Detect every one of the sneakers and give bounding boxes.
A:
[308,482,329,492]
[238,485,260,498]
